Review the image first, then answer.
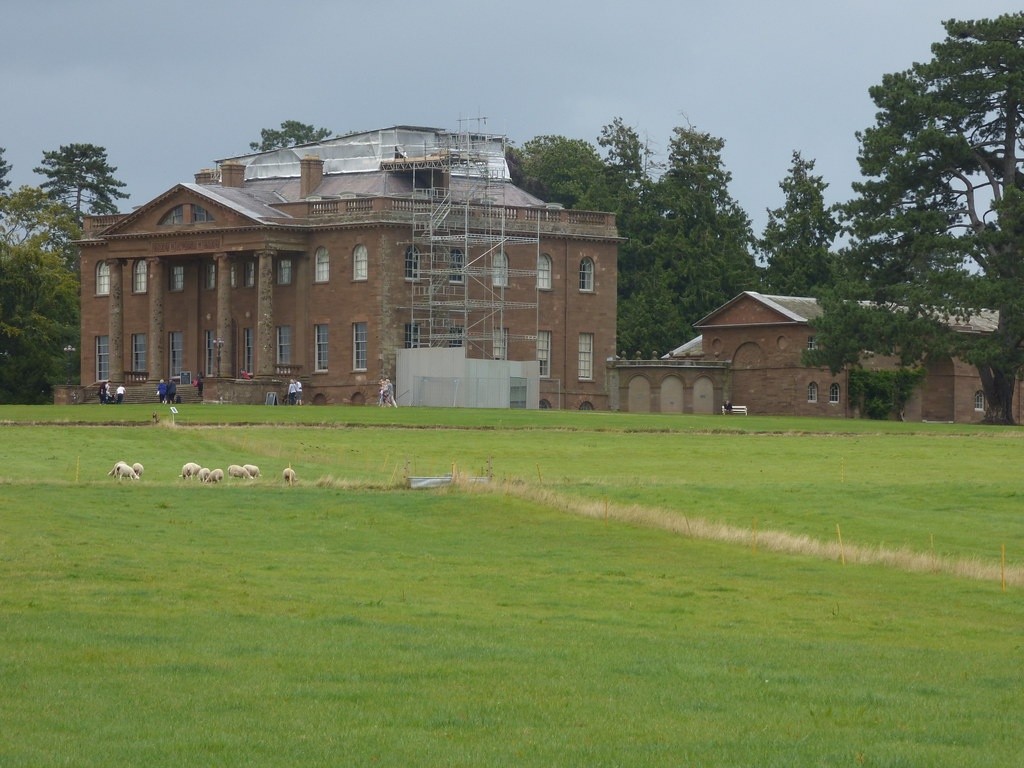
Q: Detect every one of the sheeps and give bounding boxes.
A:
[204,469,224,484]
[228,465,254,479]
[198,468,211,482]
[107,461,140,480]
[133,463,144,476]
[179,463,201,479]
[243,464,262,478]
[283,468,298,484]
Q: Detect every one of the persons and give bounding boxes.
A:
[724,400,732,414]
[158,378,167,403]
[378,378,397,408]
[242,370,250,380]
[288,378,302,405]
[99,380,112,404]
[115,384,126,404]
[196,372,204,396]
[166,378,177,404]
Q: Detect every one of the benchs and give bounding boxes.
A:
[722,406,748,416]
